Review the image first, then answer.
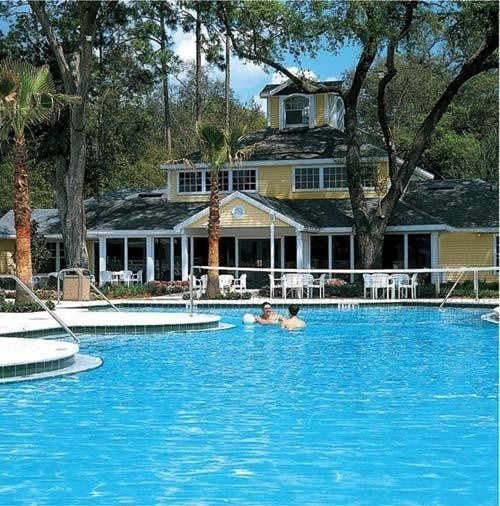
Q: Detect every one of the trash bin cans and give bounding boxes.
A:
[63,275,90,301]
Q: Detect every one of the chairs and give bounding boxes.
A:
[100,270,143,288]
[189,274,247,300]
[269,273,325,299]
[364,273,419,299]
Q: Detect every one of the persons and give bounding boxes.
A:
[280,303,306,329]
[252,302,288,324]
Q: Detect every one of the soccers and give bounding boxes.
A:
[242,313,255,326]
